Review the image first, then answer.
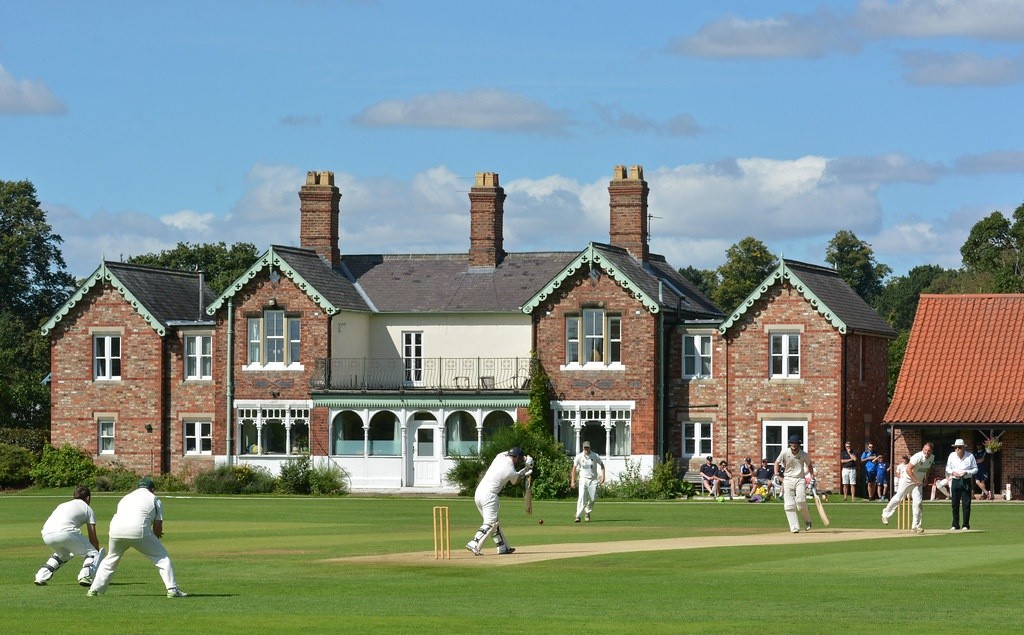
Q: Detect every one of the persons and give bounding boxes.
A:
[86,478,187,598]
[700,456,818,503]
[570,441,605,522]
[774,434,815,533]
[944,438,979,531]
[34,485,105,587]
[466,447,534,556]
[839,441,991,501]
[881,442,935,533]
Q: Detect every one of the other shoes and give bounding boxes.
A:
[843,495,886,503]
[779,495,783,500]
[980,491,992,500]
[709,493,714,496]
[805,521,811,530]
[912,527,924,532]
[951,526,967,530]
[746,494,751,498]
[792,528,799,533]
[766,492,770,496]
[881,516,889,524]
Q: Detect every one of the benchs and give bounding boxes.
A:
[682,470,707,490]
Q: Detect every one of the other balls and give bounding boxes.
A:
[537,519,544,524]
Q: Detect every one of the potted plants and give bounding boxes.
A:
[982,436,1003,454]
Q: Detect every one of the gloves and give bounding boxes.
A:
[774,475,783,485]
[810,478,816,489]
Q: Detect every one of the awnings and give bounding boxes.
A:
[40,372,52,388]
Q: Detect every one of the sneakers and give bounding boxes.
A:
[78,577,94,586]
[575,514,590,522]
[499,548,515,554]
[34,580,48,586]
[465,544,482,555]
[166,588,188,598]
[86,590,98,597]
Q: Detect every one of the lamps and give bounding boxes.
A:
[269,296,276,306]
[145,423,152,430]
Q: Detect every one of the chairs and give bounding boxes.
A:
[702,476,732,497]
[737,476,776,499]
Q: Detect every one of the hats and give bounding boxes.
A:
[951,439,966,447]
[788,435,799,443]
[746,458,751,461]
[583,441,590,447]
[707,456,712,459]
[509,448,523,458]
[138,479,157,490]
